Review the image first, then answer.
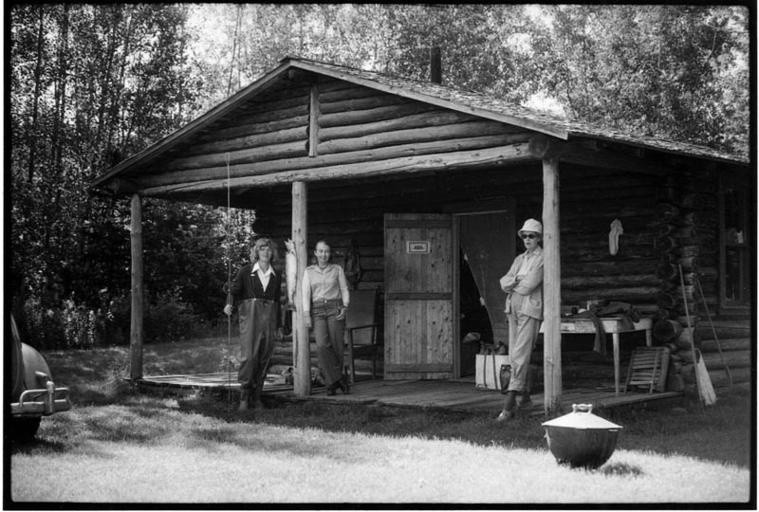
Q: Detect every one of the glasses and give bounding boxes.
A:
[522,236,537,238]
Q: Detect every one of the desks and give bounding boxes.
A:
[537,314,653,398]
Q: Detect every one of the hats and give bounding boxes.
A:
[518,218,542,236]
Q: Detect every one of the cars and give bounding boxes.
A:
[10,315,71,450]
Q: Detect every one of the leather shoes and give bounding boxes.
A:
[239,399,264,409]
[497,400,533,421]
[326,374,349,394]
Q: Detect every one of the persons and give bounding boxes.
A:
[498,218,545,422]
[301,240,351,396]
[223,237,282,412]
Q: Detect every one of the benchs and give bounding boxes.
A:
[270,295,380,383]
